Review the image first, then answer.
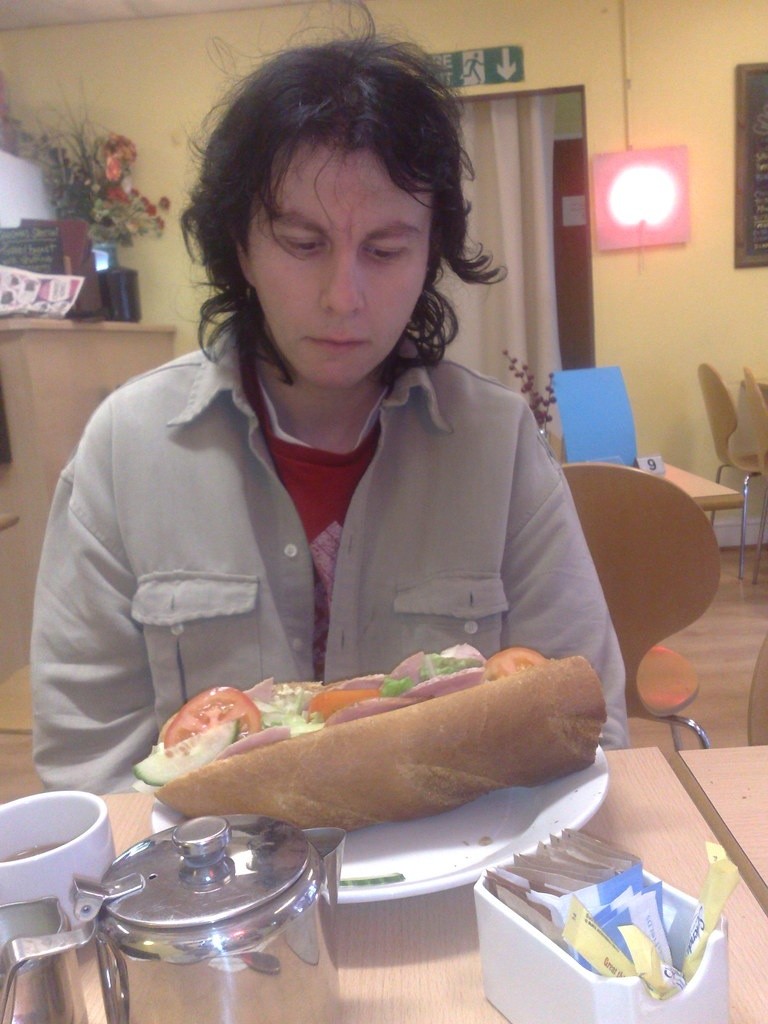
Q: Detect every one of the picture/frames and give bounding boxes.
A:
[734,62,768,268]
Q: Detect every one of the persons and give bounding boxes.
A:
[30,42,630,794]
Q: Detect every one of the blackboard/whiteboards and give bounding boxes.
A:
[0,226,66,279]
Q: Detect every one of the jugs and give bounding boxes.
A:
[0,814,347,1024]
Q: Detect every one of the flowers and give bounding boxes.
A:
[7,78,172,242]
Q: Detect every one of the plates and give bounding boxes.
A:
[150,746,610,905]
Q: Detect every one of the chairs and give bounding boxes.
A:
[698,363,761,580]
[557,464,720,751]
[744,368,768,585]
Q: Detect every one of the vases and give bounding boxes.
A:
[98,255,144,324]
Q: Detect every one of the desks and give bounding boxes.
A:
[0,744,768,1024]
[666,462,743,511]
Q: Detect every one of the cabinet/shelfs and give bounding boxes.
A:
[0,324,178,733]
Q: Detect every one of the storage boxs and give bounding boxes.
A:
[472,832,729,1024]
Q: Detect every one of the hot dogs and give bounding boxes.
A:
[129,641,609,837]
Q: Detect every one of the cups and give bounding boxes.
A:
[0,790,112,926]
[98,269,140,321]
